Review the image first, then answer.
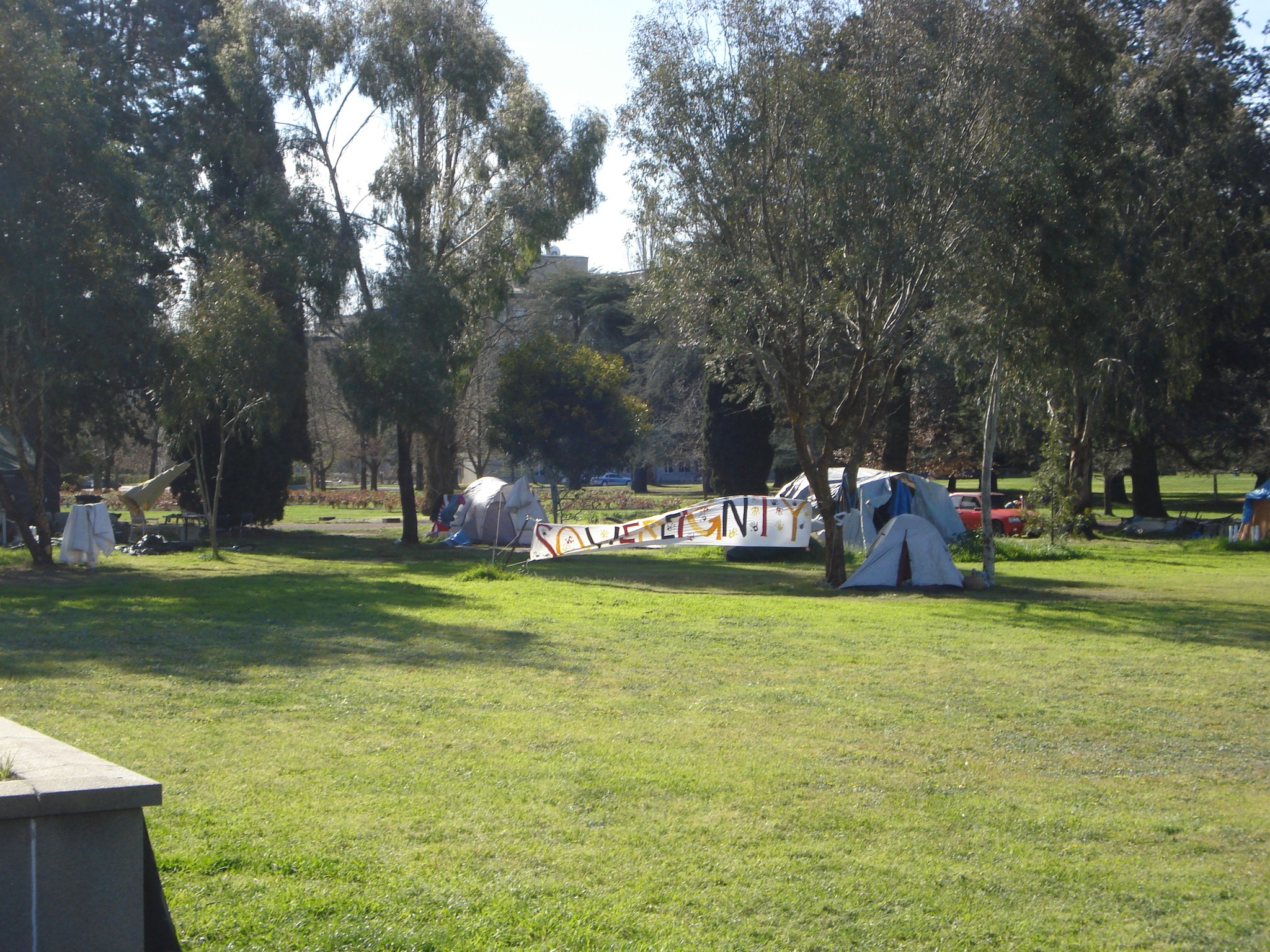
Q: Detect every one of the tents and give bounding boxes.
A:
[838,514,966,586]
[439,476,549,547]
[0,422,60,545]
[777,467,966,553]
[1236,478,1270,540]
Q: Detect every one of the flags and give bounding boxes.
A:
[430,493,465,534]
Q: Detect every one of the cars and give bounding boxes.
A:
[590,472,632,486]
[948,492,1045,538]
